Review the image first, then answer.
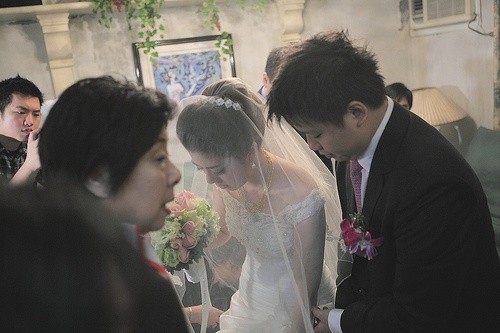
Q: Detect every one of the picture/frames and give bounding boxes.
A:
[132,34,236,115]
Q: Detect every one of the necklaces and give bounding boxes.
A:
[236,149,274,222]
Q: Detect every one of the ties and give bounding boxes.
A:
[350,159,363,216]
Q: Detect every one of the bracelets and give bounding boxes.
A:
[188,306,193,322]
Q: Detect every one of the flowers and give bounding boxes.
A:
[339,212,380,259]
[148,190,222,284]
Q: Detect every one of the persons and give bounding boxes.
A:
[184,304,225,327]
[383,82,413,112]
[0,73,45,187]
[24,74,194,333]
[149,77,354,333]
[256,43,333,176]
[261,27,500,333]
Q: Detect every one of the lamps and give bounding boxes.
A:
[408,87,466,126]
